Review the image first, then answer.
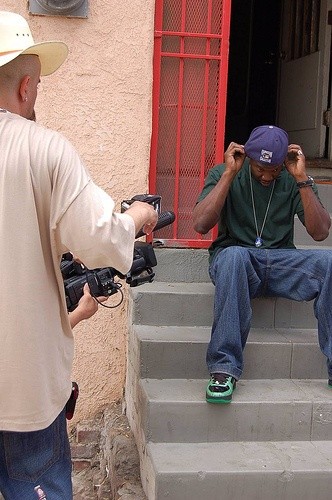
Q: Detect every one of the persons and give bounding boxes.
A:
[193,125,332,404]
[28,109,108,329]
[0,10,158,500]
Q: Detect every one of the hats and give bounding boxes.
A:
[244,125,289,168]
[0,10,70,76]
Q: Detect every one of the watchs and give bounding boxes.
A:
[296,176,314,187]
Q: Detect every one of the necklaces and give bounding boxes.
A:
[249,163,276,247]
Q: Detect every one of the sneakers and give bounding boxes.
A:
[327,379,332,389]
[205,373,237,405]
